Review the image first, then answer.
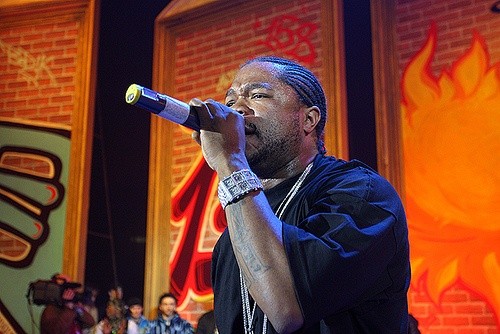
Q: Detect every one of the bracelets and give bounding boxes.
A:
[217,169,266,210]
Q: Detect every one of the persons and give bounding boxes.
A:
[39,274,95,334]
[129,303,150,329]
[212,54,422,334]
[79,285,98,334]
[197,310,220,334]
[88,299,139,334]
[147,293,195,334]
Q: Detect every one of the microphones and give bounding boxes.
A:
[63,282,81,288]
[125,84,201,132]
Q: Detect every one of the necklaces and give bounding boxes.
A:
[240,163,315,334]
